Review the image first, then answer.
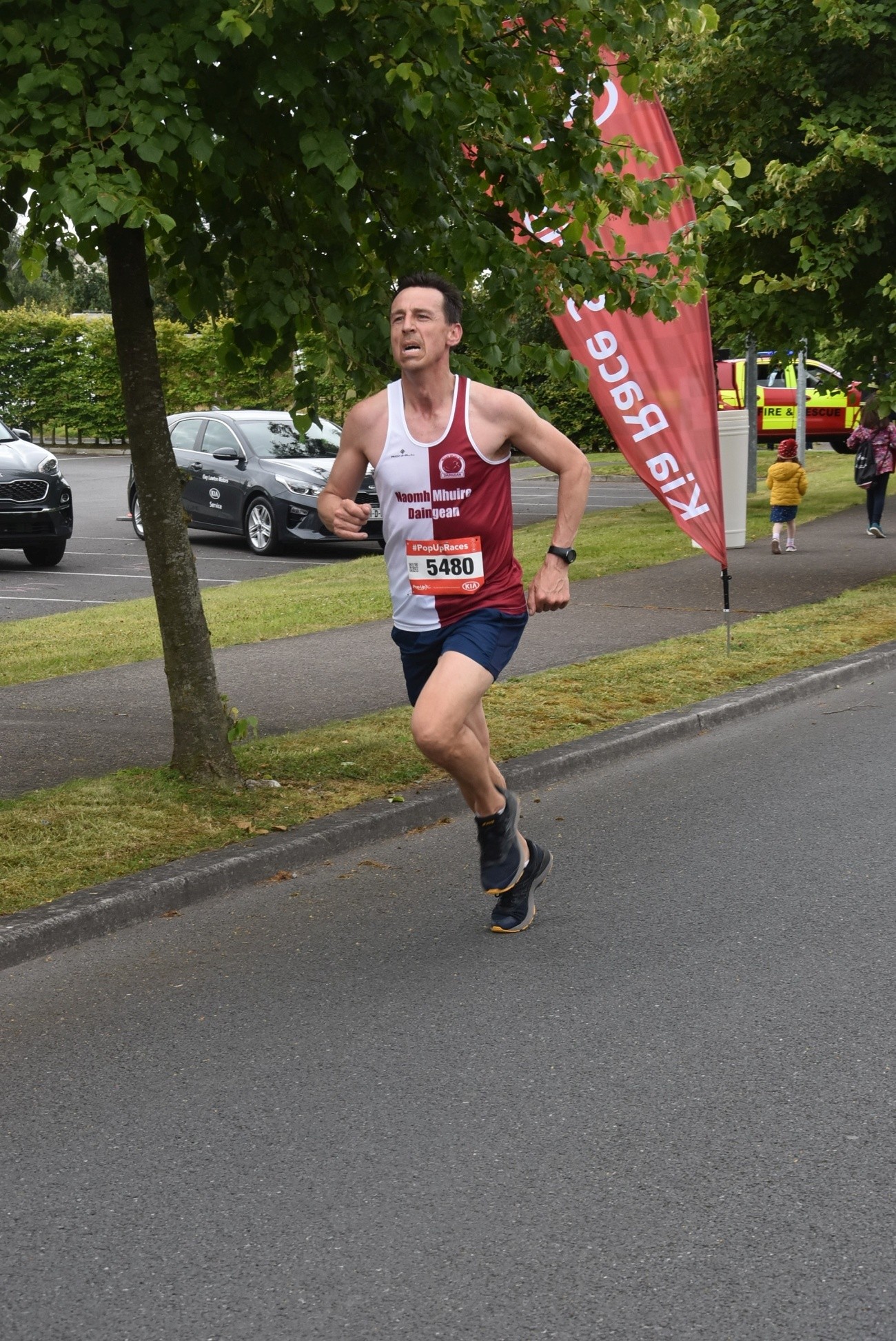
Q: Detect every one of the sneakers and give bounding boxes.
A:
[473,784,530,894]
[490,838,554,933]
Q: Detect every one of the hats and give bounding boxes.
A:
[777,438,799,459]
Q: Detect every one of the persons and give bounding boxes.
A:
[847,393,896,538]
[316,272,592,933]
[766,439,808,555]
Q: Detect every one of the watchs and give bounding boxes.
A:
[548,545,577,564]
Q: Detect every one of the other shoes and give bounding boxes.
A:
[866,527,874,536]
[772,538,782,555]
[869,522,886,538]
[785,543,798,552]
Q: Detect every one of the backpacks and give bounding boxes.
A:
[854,422,891,489]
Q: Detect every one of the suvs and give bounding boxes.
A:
[0,420,73,566]
[127,406,386,555]
[715,350,880,455]
